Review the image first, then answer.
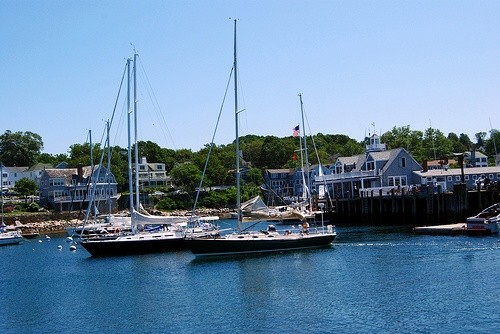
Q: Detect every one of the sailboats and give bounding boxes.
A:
[238,93,336,226]
[0,161,23,246]
[63,41,222,259]
[181,18,338,259]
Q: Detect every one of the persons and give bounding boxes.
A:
[303,221,309,233]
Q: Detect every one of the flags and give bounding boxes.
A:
[292,125,299,139]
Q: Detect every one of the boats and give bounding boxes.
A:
[6,223,40,240]
[465,201,500,238]
